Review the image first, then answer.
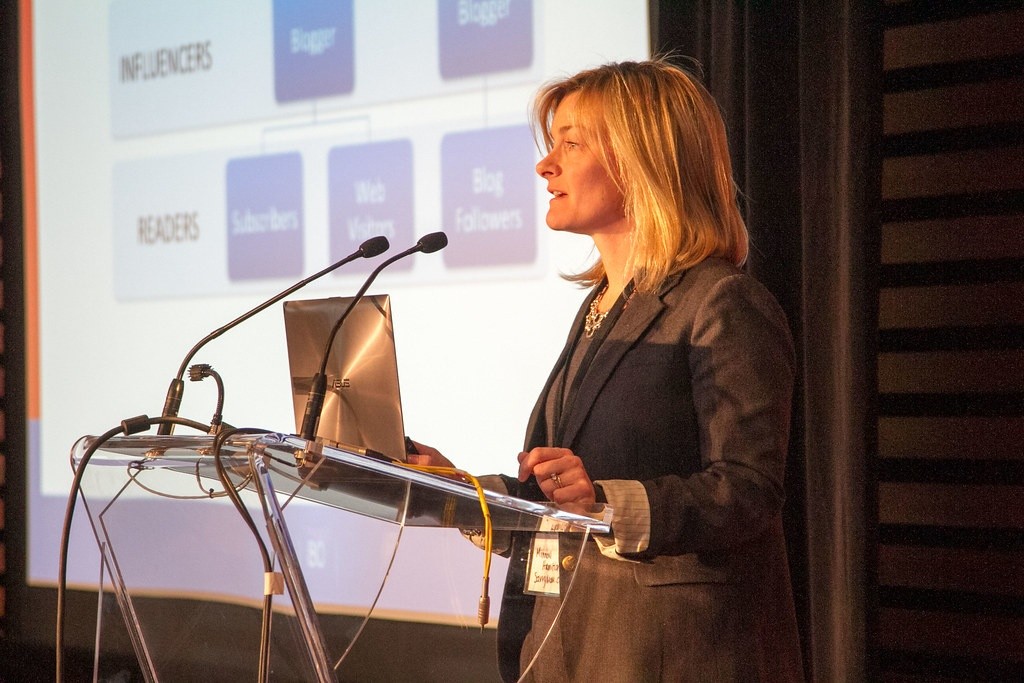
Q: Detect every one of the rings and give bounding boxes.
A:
[551,471,562,489]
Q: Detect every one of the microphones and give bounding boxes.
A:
[299,231,447,441]
[157,236,389,435]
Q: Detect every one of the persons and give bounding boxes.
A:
[379,58,806,683]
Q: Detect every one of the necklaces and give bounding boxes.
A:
[582,278,632,338]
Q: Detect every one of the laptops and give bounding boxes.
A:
[284,293,545,530]
